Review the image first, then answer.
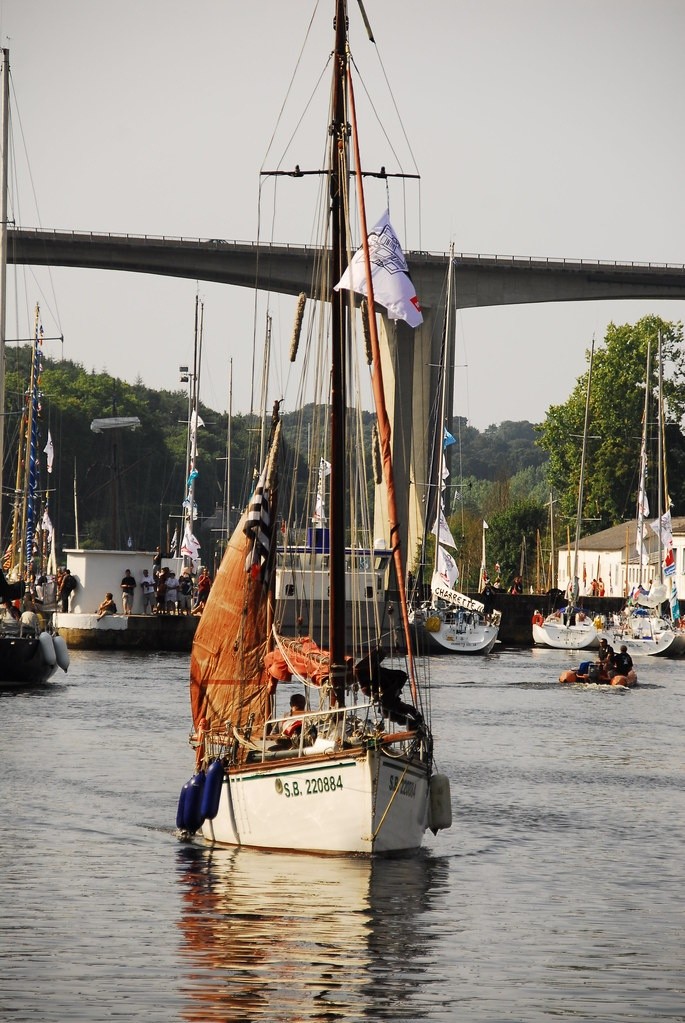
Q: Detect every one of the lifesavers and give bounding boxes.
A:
[532,613,544,627]
[196,717,207,769]
[285,720,317,750]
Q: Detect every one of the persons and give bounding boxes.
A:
[141,566,210,616]
[96,593,116,622]
[121,569,136,615]
[494,577,523,594]
[598,638,633,679]
[590,578,605,596]
[19,567,74,637]
[268,693,317,751]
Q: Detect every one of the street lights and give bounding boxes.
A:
[178,367,195,559]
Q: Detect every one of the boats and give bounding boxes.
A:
[422,561,503,657]
[559,657,638,689]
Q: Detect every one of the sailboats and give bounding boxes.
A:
[530,326,685,659]
[0,299,70,693]
[176,0,456,862]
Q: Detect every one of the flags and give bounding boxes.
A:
[431,427,459,589]
[651,512,679,618]
[42,511,52,542]
[181,408,205,561]
[334,210,424,328]
[43,431,54,472]
